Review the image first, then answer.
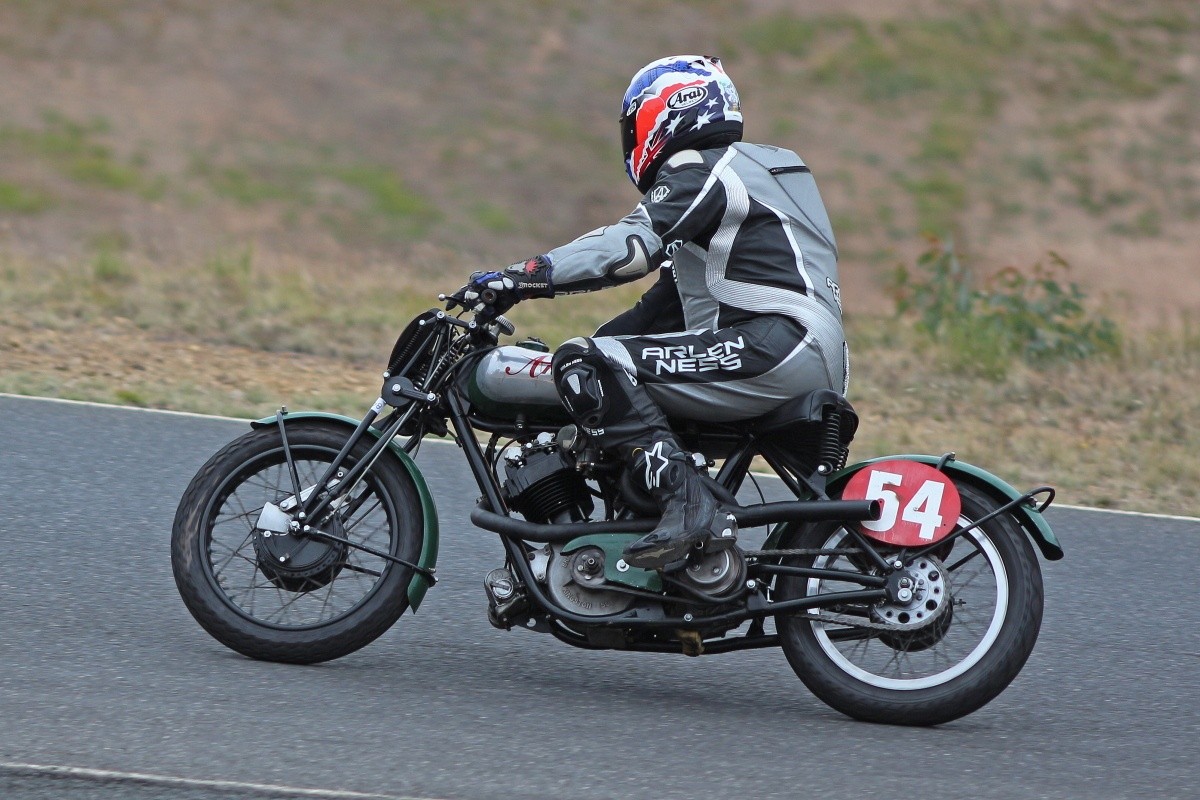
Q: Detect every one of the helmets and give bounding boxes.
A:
[619,56,743,195]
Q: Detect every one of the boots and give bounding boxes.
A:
[623,443,739,570]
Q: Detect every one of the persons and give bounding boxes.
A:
[444,55,845,571]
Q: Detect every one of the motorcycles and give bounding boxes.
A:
[170,264,1067,727]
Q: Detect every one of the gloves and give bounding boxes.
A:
[447,254,556,326]
